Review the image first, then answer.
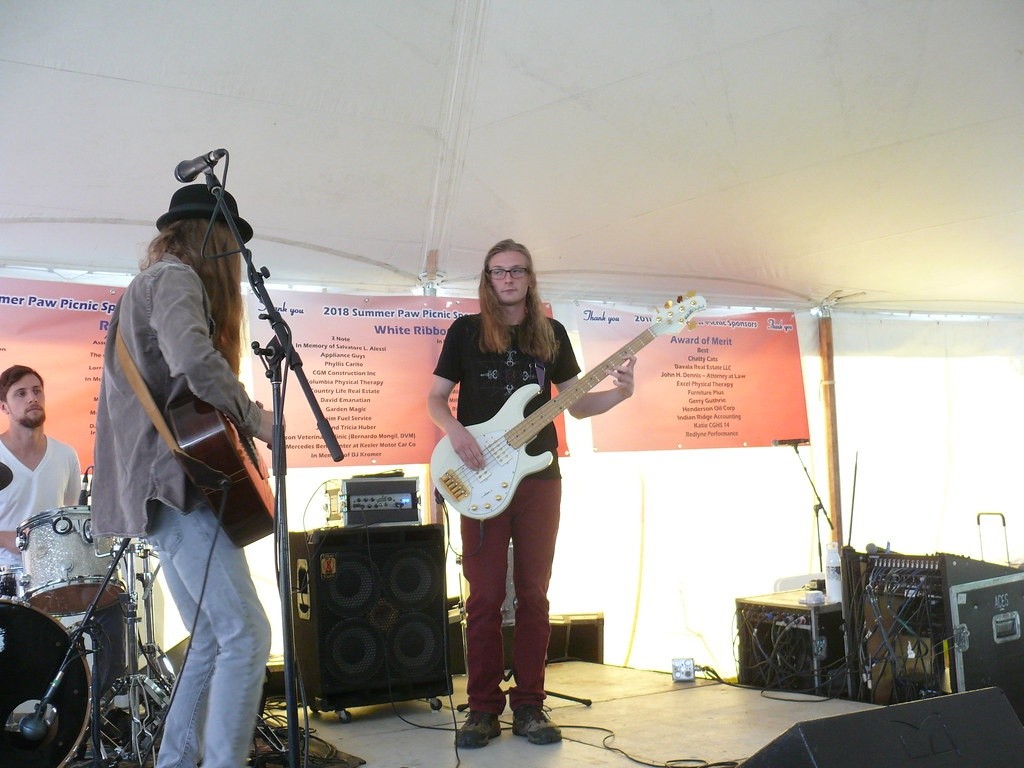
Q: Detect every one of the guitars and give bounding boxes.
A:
[168,395,276,549]
[429,290,710,521]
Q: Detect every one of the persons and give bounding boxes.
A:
[104,184,287,768]
[426,239,636,749]
[0,365,127,750]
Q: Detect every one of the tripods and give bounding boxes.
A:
[79,537,179,768]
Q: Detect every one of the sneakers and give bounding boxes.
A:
[513,706,563,745]
[454,713,502,749]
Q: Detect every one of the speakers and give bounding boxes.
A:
[290,523,454,712]
[736,686,1024,768]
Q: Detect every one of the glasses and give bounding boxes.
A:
[487,268,528,280]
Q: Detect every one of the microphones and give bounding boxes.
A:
[174,148,226,183]
[19,715,48,741]
[772,439,810,447]
[79,472,88,505]
[866,543,895,555]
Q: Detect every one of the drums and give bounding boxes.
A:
[15,505,127,617]
[0,595,92,768]
[0,565,23,601]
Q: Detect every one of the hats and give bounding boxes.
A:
[156,184,253,246]
